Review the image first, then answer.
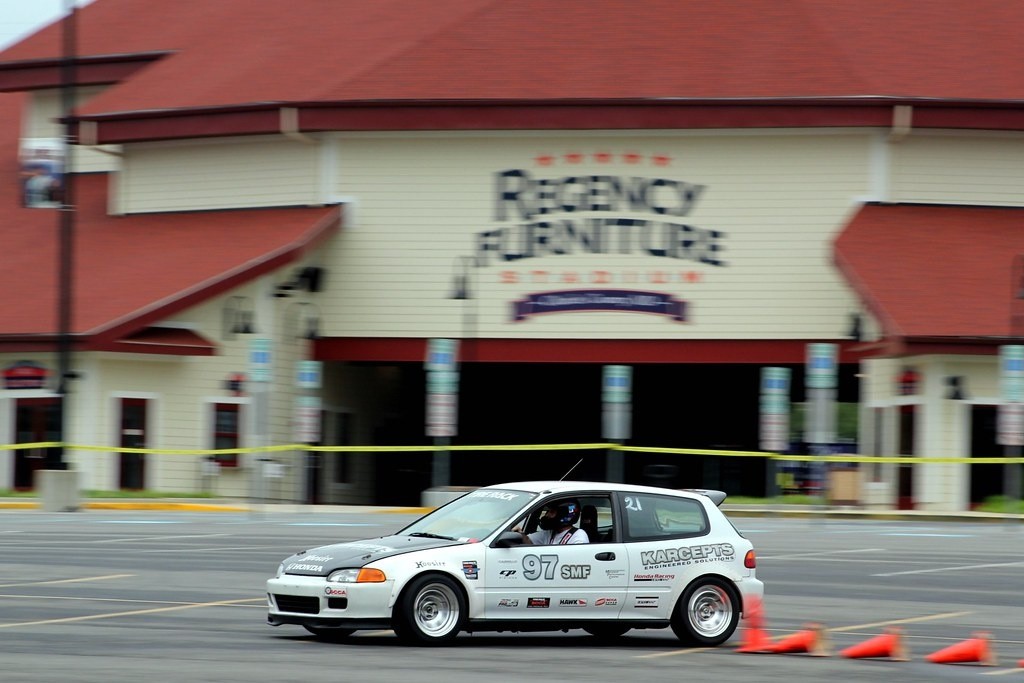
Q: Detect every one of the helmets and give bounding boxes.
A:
[538,498,581,530]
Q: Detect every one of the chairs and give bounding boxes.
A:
[580,505,602,543]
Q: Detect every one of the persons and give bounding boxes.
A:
[511,498,590,545]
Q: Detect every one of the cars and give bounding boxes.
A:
[263,480,764,648]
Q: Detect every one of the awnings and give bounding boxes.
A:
[0,327,216,362]
[835,202,1024,339]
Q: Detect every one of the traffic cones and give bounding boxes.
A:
[924,631,996,664]
[739,596,765,647]
[760,623,826,658]
[836,623,910,660]
